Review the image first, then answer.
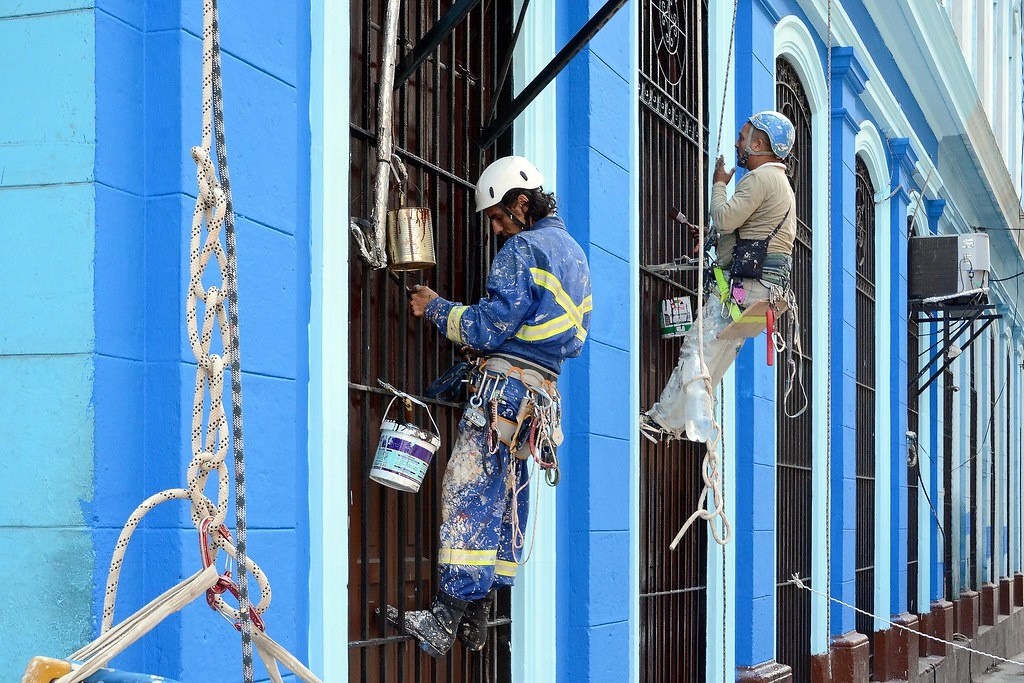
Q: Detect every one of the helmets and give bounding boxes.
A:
[475,156,545,212]
[748,111,795,160]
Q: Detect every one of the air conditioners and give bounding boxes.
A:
[908,231,990,299]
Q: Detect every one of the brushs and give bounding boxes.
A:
[670,207,695,230]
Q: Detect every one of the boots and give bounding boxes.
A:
[456,588,495,651]
[374,589,468,661]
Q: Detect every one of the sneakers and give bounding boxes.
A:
[639,407,665,444]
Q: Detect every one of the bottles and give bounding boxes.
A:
[684,351,714,443]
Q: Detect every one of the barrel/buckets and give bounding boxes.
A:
[369,392,441,493]
[386,181,435,271]
[658,296,692,338]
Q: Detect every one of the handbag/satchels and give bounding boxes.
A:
[731,239,768,280]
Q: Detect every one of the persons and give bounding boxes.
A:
[375,155,593,661]
[638,109,799,445]
